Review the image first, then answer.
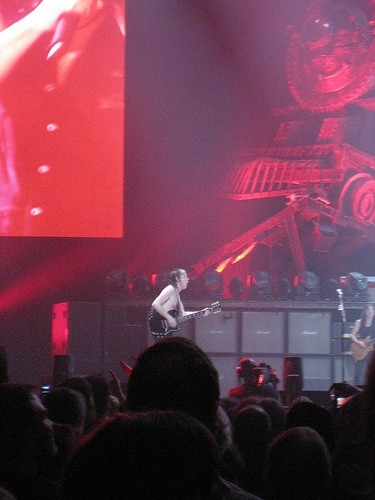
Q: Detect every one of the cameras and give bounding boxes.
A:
[40,384,53,394]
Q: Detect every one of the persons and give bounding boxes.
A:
[148,268,210,343]
[351,304,375,386]
[0,0,113,236]
[0,336,375,500]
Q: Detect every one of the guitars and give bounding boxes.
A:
[351,335,375,360]
[149,301,221,339]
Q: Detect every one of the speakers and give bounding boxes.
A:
[284,356,303,391]
[52,354,73,385]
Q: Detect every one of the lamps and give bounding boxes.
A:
[250,271,274,301]
[293,272,321,301]
[344,272,370,303]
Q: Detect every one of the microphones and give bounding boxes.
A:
[336,289,343,298]
